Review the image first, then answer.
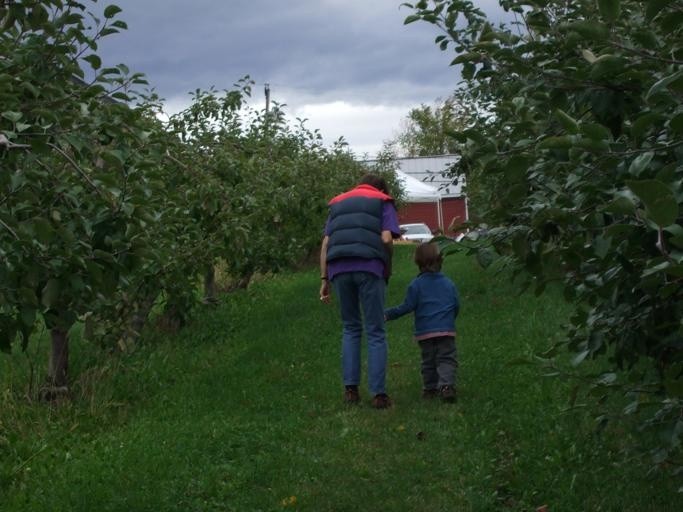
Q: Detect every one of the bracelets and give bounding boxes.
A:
[319,276,329,282]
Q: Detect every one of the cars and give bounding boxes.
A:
[397,220,434,244]
[452,229,474,243]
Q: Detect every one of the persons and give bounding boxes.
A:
[319,173,401,409]
[384,243,460,403]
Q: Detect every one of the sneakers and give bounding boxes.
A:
[373,394,391,408]
[440,387,456,403]
[422,388,436,399]
[344,388,360,402]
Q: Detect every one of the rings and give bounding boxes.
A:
[320,297,324,300]
